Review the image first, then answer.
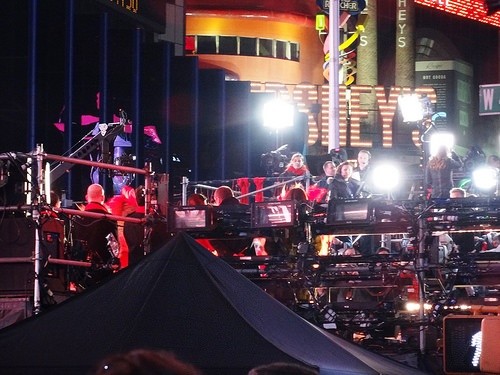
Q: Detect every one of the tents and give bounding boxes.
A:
[0,231,434,375]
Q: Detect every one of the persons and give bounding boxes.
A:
[353,150,372,182]
[447,187,475,253]
[71,184,120,293]
[39,191,66,262]
[263,188,322,301]
[328,162,361,200]
[274,153,316,201]
[358,247,406,310]
[335,247,368,303]
[313,161,337,204]
[209,186,265,292]
[425,145,465,198]
[123,187,171,267]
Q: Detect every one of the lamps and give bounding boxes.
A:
[169,196,372,233]
